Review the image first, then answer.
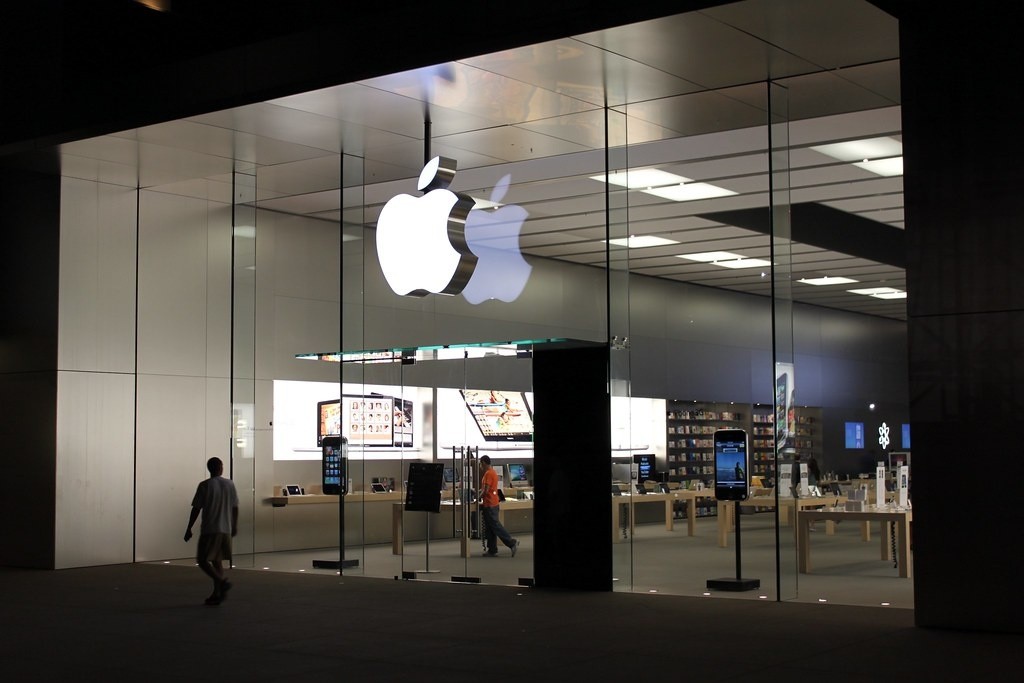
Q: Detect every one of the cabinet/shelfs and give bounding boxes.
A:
[667,399,824,517]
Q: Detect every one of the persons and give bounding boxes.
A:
[790,453,801,499]
[807,458,821,532]
[351,401,389,433]
[460,457,483,539]
[184,457,239,607]
[488,391,521,428]
[394,410,406,426]
[473,455,520,557]
[735,462,745,480]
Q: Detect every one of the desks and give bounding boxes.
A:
[394,476,913,580]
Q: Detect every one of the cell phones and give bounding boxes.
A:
[322,437,348,495]
[714,429,749,501]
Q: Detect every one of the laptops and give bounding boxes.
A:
[440,391,533,450]
[295,391,421,453]
[457,488,476,504]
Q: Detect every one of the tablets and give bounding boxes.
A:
[286,485,302,496]
[750,487,757,498]
[659,484,670,493]
[688,479,700,490]
[611,484,622,495]
[635,484,646,494]
[371,483,387,493]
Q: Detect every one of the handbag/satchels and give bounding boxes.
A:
[497,489,506,502]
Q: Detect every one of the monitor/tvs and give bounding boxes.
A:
[507,463,528,485]
[443,467,461,487]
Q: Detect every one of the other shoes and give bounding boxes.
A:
[205,590,227,605]
[482,550,498,556]
[511,540,520,557]
[219,577,233,601]
